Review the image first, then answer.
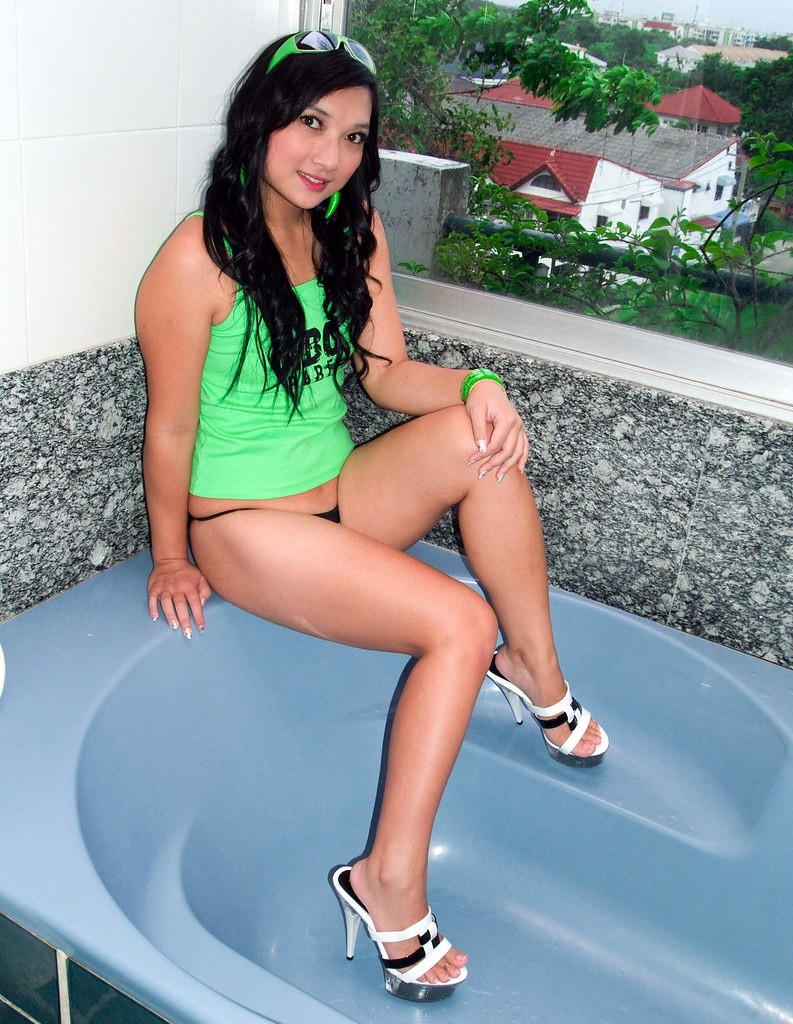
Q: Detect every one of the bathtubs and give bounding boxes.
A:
[1,535,793,1024]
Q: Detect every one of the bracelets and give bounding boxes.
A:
[460,368,505,402]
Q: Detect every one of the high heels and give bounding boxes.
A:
[485,650,609,768]
[333,866,467,1002]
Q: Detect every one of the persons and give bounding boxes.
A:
[135,30,610,1001]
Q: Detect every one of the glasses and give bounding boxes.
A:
[265,30,378,78]
